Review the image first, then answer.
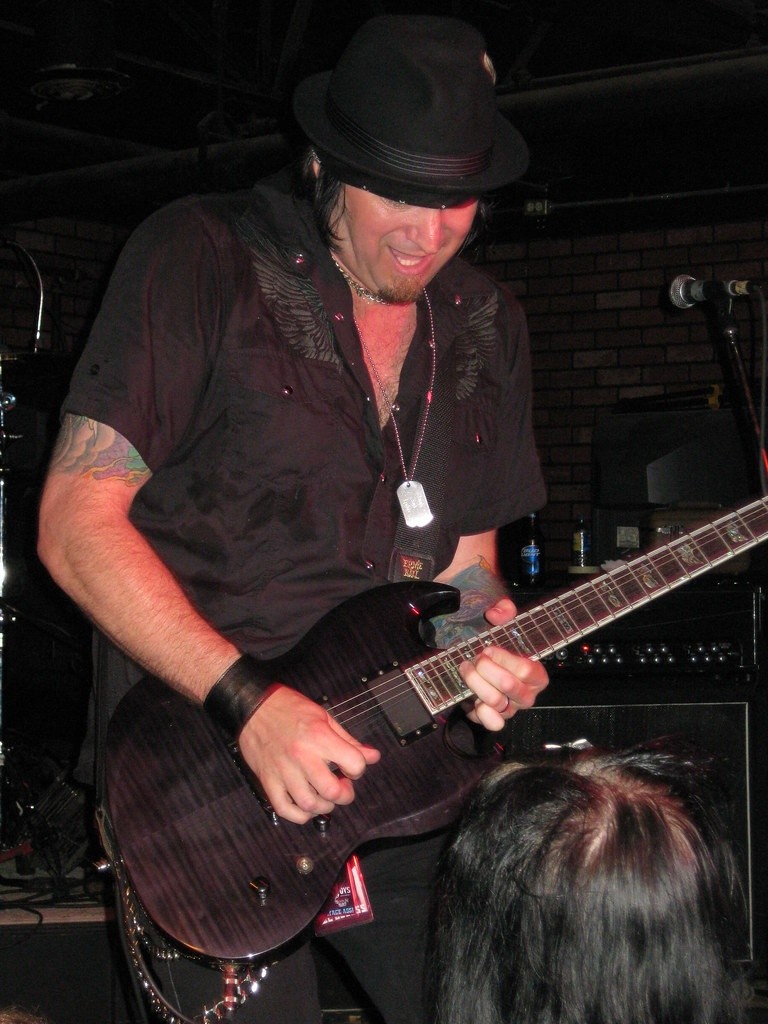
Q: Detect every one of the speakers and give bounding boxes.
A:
[468,577,768,997]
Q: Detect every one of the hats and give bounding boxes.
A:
[295,15,531,206]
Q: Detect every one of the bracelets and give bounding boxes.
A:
[202,652,287,740]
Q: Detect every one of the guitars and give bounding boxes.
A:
[100,491,768,964]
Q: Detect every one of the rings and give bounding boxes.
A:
[498,696,510,713]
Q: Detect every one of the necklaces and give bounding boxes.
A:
[352,287,438,529]
[332,255,391,306]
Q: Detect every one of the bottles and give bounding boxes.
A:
[573,519,592,566]
[520,512,544,588]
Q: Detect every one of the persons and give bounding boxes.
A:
[419,744,753,1024]
[35,15,551,1024]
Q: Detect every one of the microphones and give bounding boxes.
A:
[670,274,758,309]
